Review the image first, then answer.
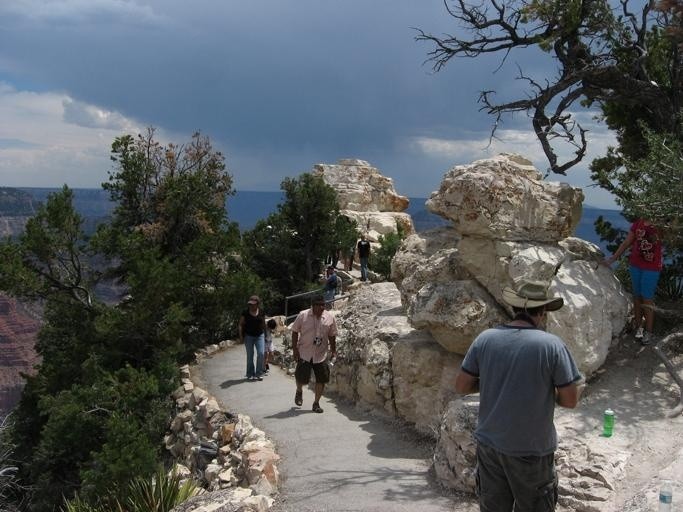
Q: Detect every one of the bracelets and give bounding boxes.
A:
[615,253,618,258]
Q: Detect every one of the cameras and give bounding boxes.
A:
[316,339,321,347]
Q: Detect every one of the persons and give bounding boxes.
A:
[602,202,663,346]
[264,318,277,373]
[456,281,580,512]
[324,236,370,311]
[238,296,267,381]
[289,293,339,413]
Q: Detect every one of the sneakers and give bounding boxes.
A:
[294,389,303,406]
[312,401,323,413]
[244,364,271,381]
[633,326,656,345]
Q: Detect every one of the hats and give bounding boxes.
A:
[246,294,260,305]
[502,279,564,312]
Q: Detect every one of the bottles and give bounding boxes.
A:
[658,478,672,512]
[602,407,614,439]
